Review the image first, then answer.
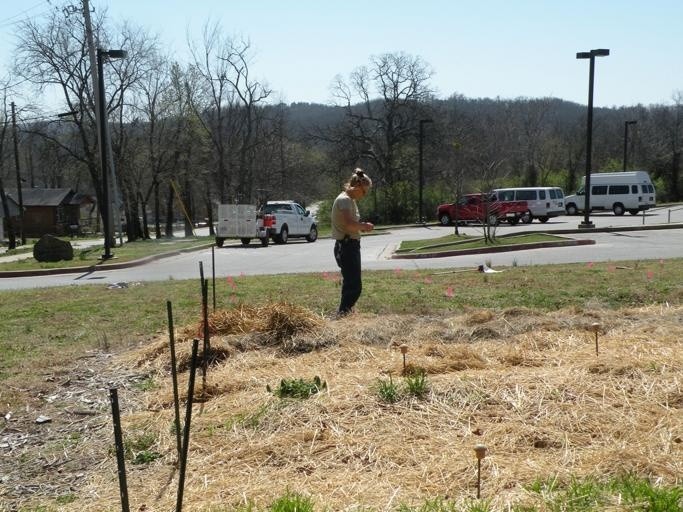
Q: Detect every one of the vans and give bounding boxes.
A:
[490,187,565,224]
[563,172,656,215]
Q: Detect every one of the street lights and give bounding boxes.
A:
[97,47,127,257]
[420,119,431,221]
[623,121,636,171]
[575,48,608,223]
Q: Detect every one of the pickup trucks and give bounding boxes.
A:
[242,199,317,246]
[436,193,527,226]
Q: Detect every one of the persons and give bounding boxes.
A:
[331,168,375,317]
[256,196,264,215]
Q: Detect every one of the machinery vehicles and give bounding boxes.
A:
[214,203,269,246]
